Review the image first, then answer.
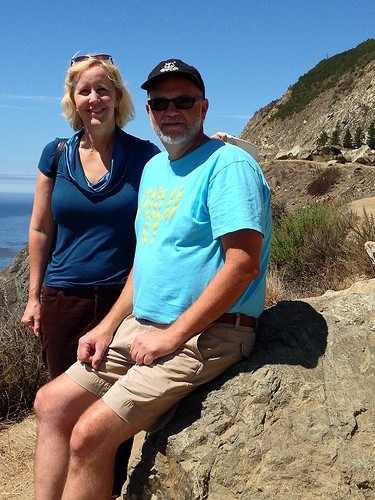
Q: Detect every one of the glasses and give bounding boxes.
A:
[70,53,114,68]
[147,95,203,112]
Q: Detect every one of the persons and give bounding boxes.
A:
[21,52,227,493]
[30,57,272,499]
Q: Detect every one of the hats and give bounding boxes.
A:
[140,58,206,98]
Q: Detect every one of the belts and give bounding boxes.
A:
[216,312,258,330]
